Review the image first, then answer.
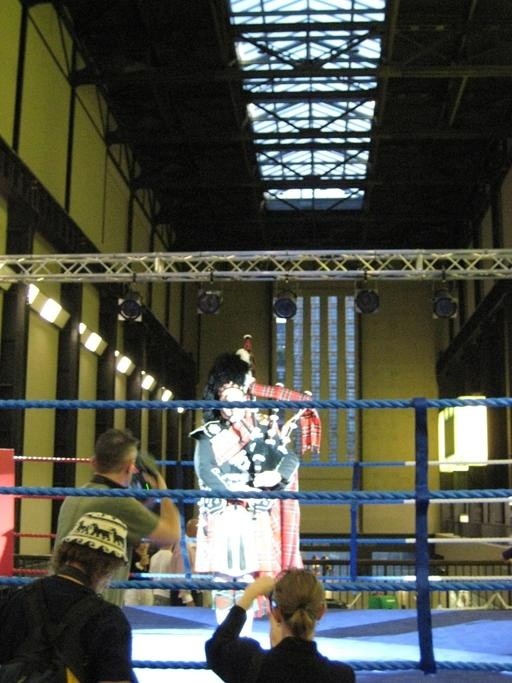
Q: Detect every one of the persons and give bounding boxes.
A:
[50,431,181,608]
[206,567,356,683]
[189,386,299,636]
[0,512,139,683]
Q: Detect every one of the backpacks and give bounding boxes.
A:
[0,595,111,683]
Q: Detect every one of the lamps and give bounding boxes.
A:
[196,269,224,315]
[355,269,380,314]
[433,268,457,318]
[120,272,142,323]
[436,369,488,473]
[273,273,299,319]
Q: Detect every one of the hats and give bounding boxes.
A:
[61,511,129,563]
